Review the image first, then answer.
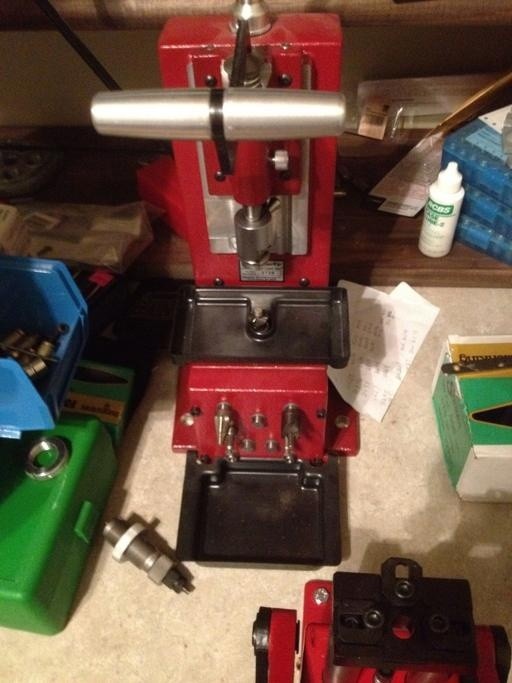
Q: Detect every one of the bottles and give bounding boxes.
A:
[416,161,466,259]
